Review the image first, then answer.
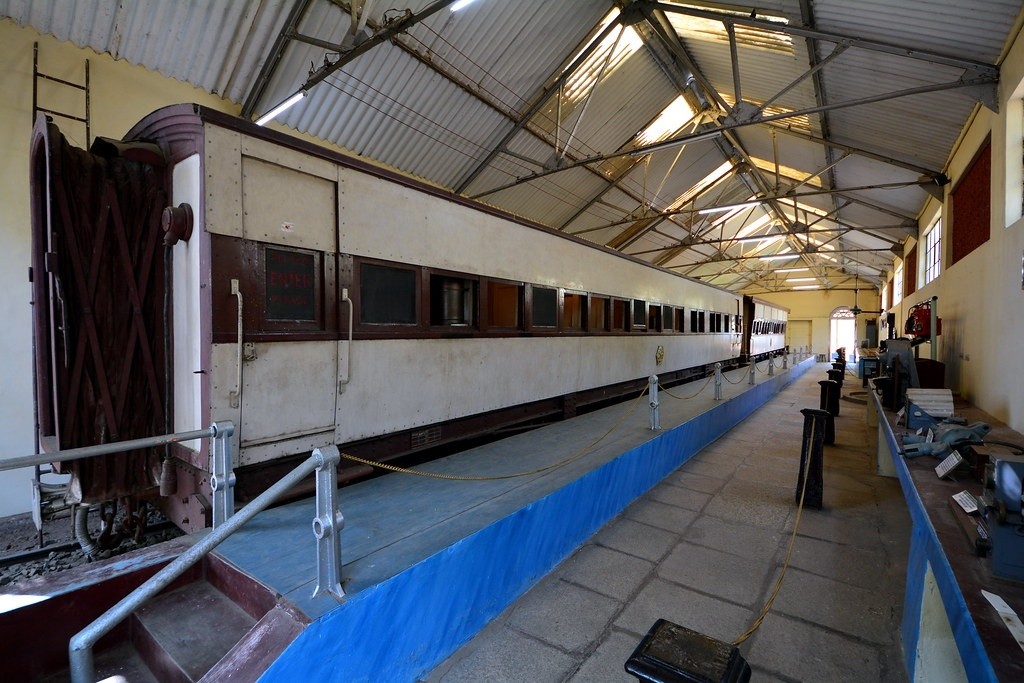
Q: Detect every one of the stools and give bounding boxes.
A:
[818,354,826,363]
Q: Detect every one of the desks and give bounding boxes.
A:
[857,348,882,387]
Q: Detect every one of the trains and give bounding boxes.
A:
[29,98,791,559]
